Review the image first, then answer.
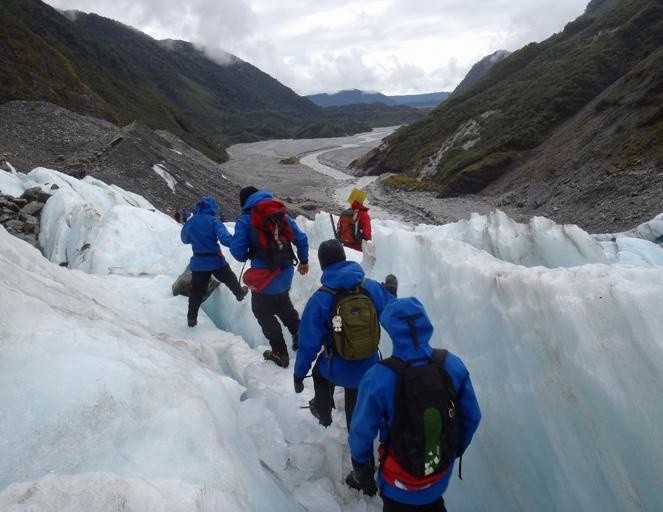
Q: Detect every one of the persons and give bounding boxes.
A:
[175,208,225,223]
[337,201,371,252]
[348,297,481,512]
[230,186,308,368]
[293,239,397,431]
[181,195,247,327]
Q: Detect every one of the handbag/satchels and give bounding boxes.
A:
[243,265,286,291]
[378,447,447,490]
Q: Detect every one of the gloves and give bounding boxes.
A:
[381,275,397,296]
[293,375,304,393]
[352,460,371,485]
[336,209,364,244]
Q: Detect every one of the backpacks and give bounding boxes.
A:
[244,201,295,263]
[380,349,459,476]
[318,280,380,362]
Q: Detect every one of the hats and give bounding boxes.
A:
[239,186,258,206]
[318,239,346,270]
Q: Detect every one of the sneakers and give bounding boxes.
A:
[187,320,196,326]
[264,350,288,367]
[237,286,247,301]
[346,473,378,496]
[292,335,299,349]
[309,399,332,424]
